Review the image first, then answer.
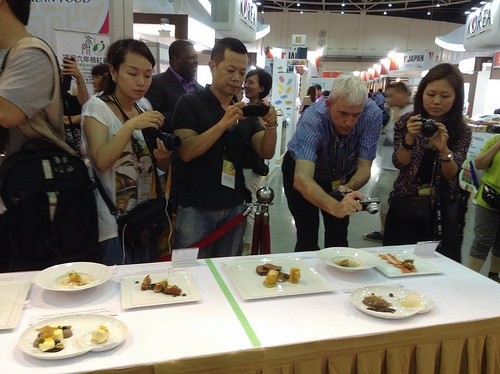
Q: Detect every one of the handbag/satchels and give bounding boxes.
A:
[482,184,500,208]
[119,198,173,264]
[0,138,98,273]
[390,196,435,245]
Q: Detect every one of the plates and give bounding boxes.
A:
[18,312,130,360]
[119,269,200,311]
[371,250,445,279]
[221,256,338,301]
[35,262,112,293]
[316,246,378,271]
[0,278,32,330]
[350,283,426,319]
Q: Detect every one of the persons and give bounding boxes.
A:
[298,84,330,113]
[282,74,384,252]
[0,0,67,273]
[384,63,473,265]
[82,39,171,268]
[171,38,277,260]
[469,134,500,283]
[367,89,391,135]
[144,40,205,198]
[362,81,414,242]
[58,54,109,265]
[232,67,272,256]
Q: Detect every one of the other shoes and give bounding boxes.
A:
[362,231,383,241]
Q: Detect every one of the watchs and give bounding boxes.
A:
[438,150,454,162]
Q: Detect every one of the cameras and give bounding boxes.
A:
[243,104,269,116]
[155,127,182,151]
[416,118,438,136]
[357,197,380,213]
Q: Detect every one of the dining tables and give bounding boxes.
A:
[0,244,500,374]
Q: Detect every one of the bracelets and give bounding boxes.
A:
[264,120,279,129]
[75,79,85,85]
[401,138,417,150]
